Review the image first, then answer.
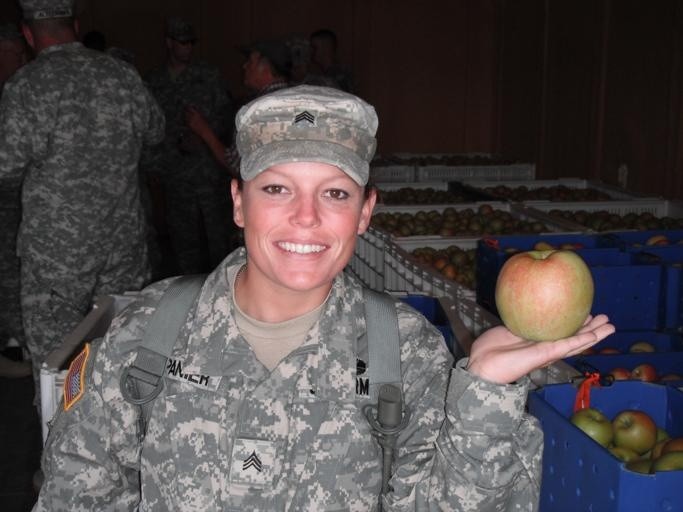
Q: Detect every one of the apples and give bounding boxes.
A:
[494,248,595,341]
[569,405,682,473]
[372,155,683,381]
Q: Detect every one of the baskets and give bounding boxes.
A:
[344,153,682,511]
[349,163,682,510]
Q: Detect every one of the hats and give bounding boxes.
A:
[236,85,379,186]
[167,22,202,41]
[235,36,292,66]
[20,0,72,19]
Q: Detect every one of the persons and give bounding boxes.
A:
[2,4,355,482]
[34,85,618,512]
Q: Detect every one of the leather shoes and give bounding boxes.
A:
[1,352,31,380]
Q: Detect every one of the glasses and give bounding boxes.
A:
[170,37,198,47]
